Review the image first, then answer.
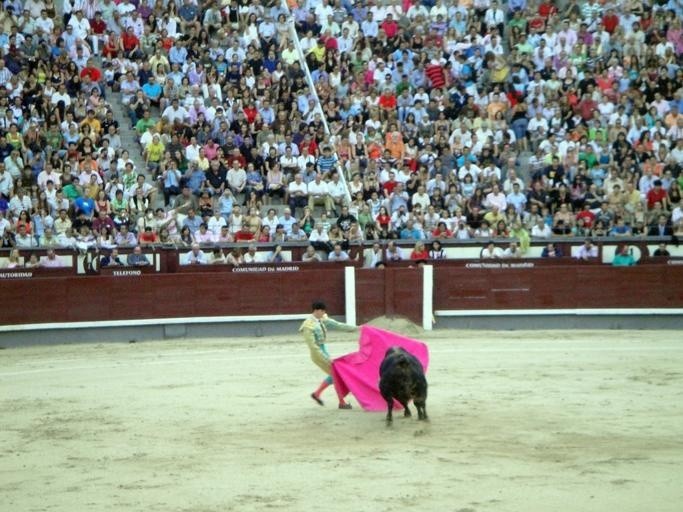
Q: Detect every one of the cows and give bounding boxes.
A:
[378,344,429,428]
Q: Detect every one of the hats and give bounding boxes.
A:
[312,300,327,310]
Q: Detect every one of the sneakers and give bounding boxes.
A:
[312,392,324,407]
[339,402,353,409]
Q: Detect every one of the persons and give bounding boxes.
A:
[1,0,683,277]
[298,301,361,410]
